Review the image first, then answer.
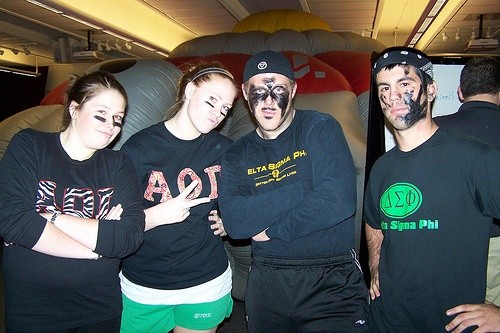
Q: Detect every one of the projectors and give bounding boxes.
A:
[73,51,104,62]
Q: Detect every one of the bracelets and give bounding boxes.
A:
[49,213,58,224]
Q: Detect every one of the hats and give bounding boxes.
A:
[242,50,294,83]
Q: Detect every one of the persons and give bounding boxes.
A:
[360,45,500,333]
[219,51,369,333]
[118,61,237,333]
[431,55,500,308]
[0,71,147,333]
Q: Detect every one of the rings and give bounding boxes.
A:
[186,208,190,211]
